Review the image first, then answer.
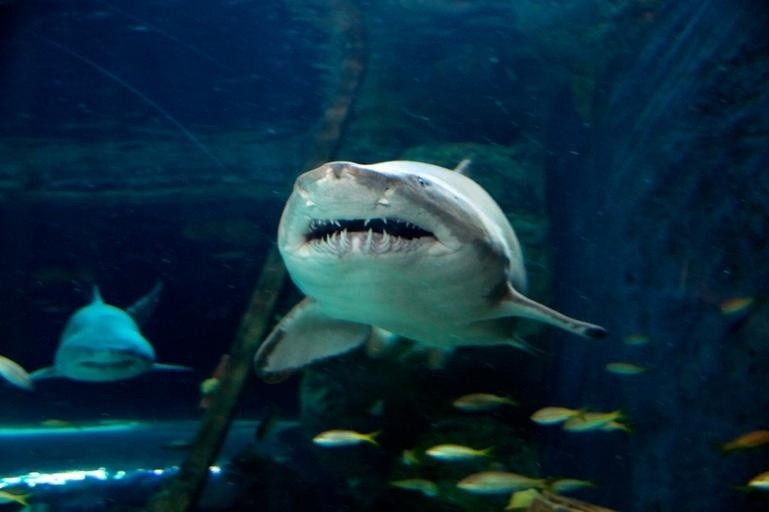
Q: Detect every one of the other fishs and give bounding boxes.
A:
[0,356,31,391]
[731,471,769,496]
[311,428,383,449]
[200,377,219,396]
[255,401,282,441]
[40,419,81,429]
[713,429,769,454]
[606,362,648,375]
[392,393,632,511]
[0,487,33,508]
[624,334,652,344]
[721,295,761,314]
